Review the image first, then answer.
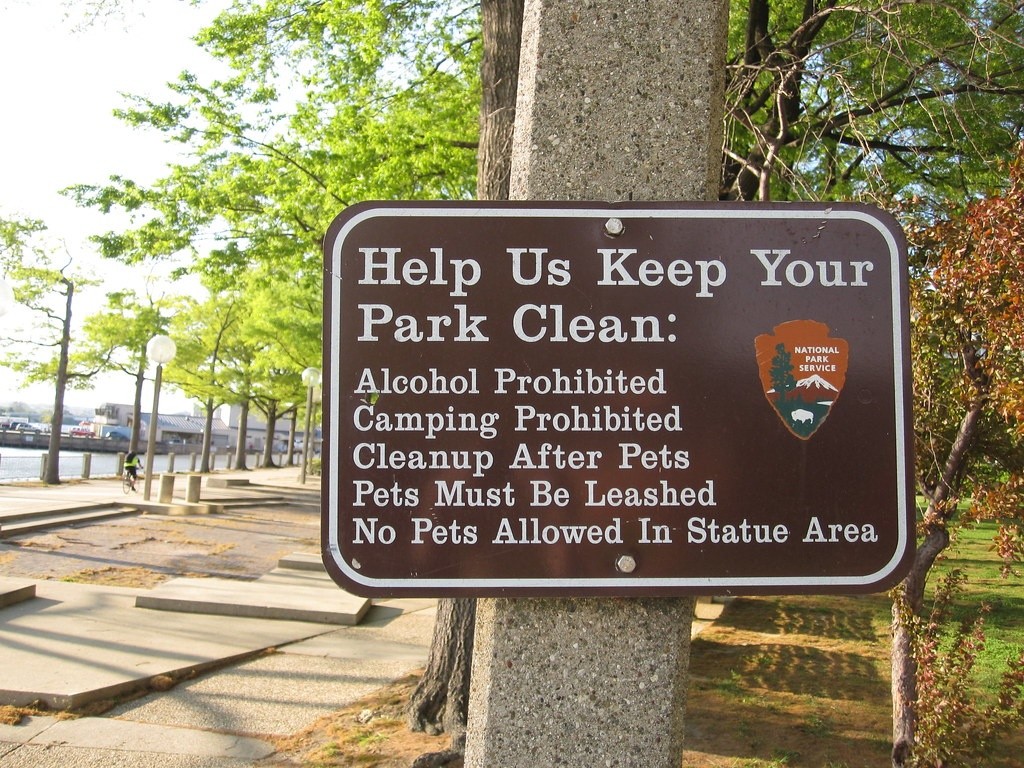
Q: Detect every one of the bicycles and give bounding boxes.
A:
[122,466,144,494]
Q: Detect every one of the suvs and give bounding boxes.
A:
[68,426,96,438]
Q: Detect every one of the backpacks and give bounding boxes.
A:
[126,454,136,463]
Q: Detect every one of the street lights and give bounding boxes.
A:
[142,334,177,499]
[300,366,323,485]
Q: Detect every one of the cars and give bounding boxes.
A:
[105,428,131,441]
[0,421,42,434]
[166,438,192,445]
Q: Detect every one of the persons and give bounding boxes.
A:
[124,450,143,491]
[210,441,217,452]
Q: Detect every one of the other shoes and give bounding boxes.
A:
[132,487,135,490]
